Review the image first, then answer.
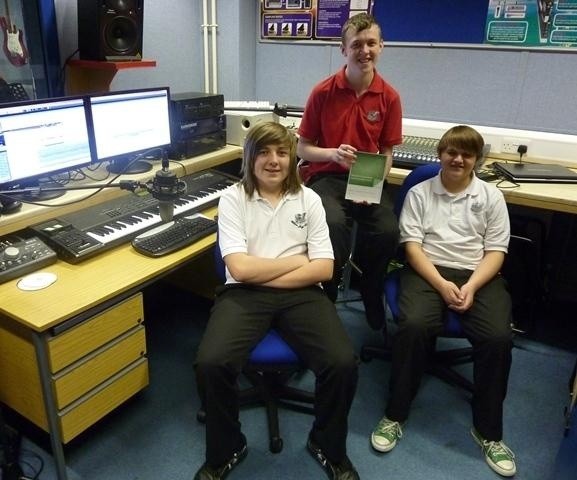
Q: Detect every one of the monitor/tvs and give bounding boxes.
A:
[0,95,94,202]
[90,86,172,174]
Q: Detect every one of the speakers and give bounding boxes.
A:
[77,0,144,62]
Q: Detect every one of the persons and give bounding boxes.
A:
[294,10,404,333]
[371,126,518,476]
[195,123,360,480]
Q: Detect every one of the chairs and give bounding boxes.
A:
[359,162,520,393]
[198,228,315,455]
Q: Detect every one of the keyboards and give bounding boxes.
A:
[131,215,218,258]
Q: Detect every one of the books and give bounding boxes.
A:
[343,145,386,204]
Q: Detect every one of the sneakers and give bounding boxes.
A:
[194,438,248,480]
[470,424,517,477]
[371,414,409,453]
[307,436,360,480]
[361,284,386,329]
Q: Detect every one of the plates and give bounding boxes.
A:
[16,272,58,291]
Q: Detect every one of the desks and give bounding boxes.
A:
[386,154,577,215]
[1,144,243,480]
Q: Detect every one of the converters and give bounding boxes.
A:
[517,145,527,154]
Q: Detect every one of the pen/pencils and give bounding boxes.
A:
[347,149,353,153]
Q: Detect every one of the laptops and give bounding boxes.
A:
[493,161,577,185]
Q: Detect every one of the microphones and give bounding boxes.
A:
[151,150,181,223]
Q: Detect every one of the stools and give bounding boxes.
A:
[297,157,368,305]
